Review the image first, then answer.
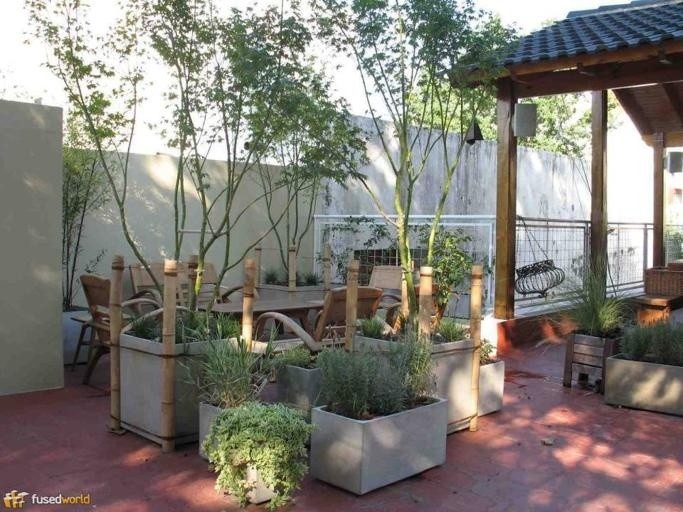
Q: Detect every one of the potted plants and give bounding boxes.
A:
[478,339,507,417]
[19,1,370,454]
[278,0,513,437]
[202,401,322,511]
[315,330,447,497]
[175,302,282,463]
[539,245,635,397]
[275,343,324,449]
[603,317,683,416]
